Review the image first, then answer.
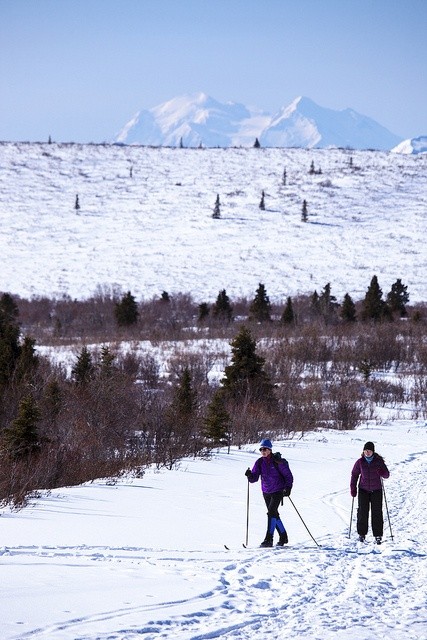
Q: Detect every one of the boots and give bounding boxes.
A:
[276,532,288,546]
[260,534,273,547]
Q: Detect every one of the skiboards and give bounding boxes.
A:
[223,544,322,550]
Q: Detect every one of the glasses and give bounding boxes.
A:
[259,448,266,452]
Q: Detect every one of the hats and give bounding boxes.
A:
[260,439,272,450]
[364,442,374,451]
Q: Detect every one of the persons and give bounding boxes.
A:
[244,437,295,549]
[349,442,391,545]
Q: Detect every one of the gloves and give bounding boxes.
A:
[350,487,357,497]
[281,488,290,496]
[244,469,252,479]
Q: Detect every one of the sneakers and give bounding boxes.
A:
[374,536,381,545]
[356,537,364,542]
